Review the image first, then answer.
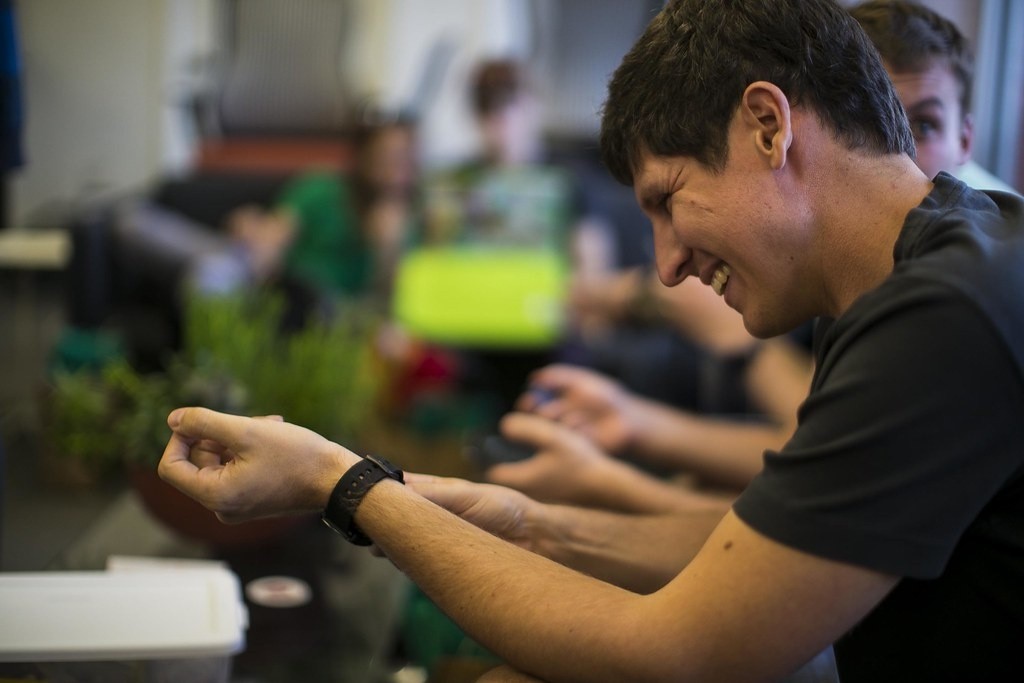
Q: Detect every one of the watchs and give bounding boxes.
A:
[323,452,405,548]
[725,335,764,378]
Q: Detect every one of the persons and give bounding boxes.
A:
[471,1,973,518]
[157,0,1024,682]
[644,270,818,423]
[469,57,622,336]
[345,111,434,255]
